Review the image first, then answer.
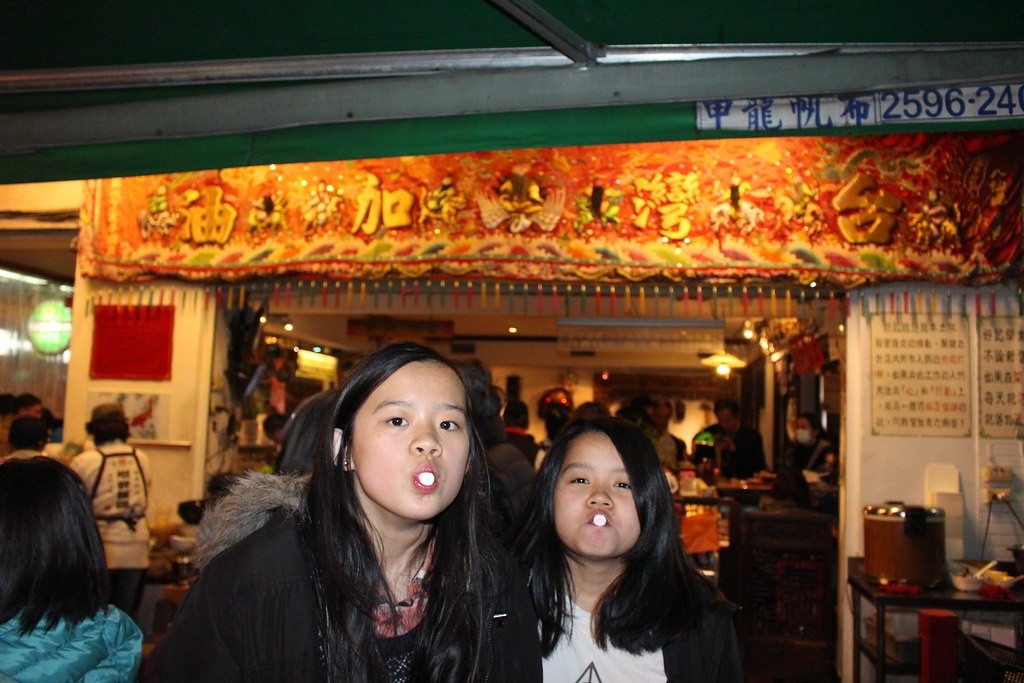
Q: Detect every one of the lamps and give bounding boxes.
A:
[557,317,726,328]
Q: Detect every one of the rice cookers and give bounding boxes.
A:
[863,498,945,582]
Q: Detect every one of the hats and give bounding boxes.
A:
[85,405,126,434]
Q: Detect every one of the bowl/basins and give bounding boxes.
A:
[177,500,205,524]
[171,535,195,552]
[950,568,1014,591]
[1007,544,1024,564]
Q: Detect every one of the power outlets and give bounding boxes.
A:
[986,487,1012,504]
[986,467,1013,481]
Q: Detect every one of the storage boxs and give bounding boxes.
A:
[863,618,1024,682]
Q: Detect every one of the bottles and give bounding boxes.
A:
[679,469,697,495]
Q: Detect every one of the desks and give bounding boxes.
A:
[848,570,1024,683]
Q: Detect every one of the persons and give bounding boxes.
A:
[0,390,837,683]
[505,417,740,683]
[157,341,514,683]
